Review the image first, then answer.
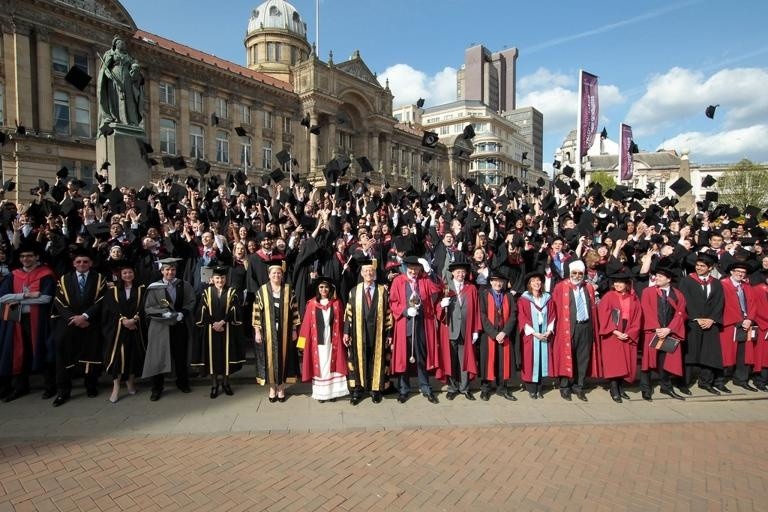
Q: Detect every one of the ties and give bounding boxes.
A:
[366,288,371,307]
[167,285,174,304]
[662,290,667,311]
[703,286,708,299]
[80,277,86,295]
[736,285,746,312]
[575,286,585,320]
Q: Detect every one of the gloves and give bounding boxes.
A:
[470,333,478,346]
[441,297,450,308]
[163,310,182,322]
[406,293,421,316]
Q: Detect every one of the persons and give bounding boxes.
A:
[97,33,146,128]
[2,176,768,407]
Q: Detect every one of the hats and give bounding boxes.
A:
[4,97,762,284]
[63,65,95,92]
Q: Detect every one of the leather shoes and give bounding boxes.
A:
[0,367,768,406]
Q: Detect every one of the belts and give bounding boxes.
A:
[576,321,589,326]
